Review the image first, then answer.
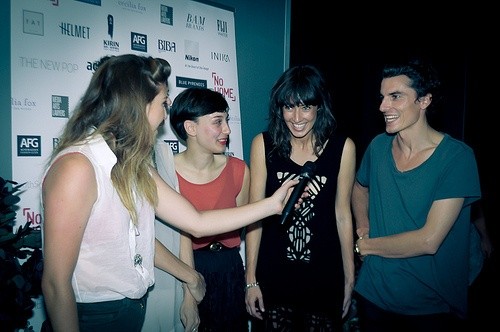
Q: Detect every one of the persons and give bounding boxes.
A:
[169,86,251,332]
[244,65,356,332]
[41,54,310,332]
[141,141,201,332]
[352,61,492,332]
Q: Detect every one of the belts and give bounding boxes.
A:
[208,242,223,252]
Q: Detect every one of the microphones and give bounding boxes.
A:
[281,160,318,226]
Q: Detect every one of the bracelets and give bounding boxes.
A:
[355,236,366,257]
[244,281,259,291]
